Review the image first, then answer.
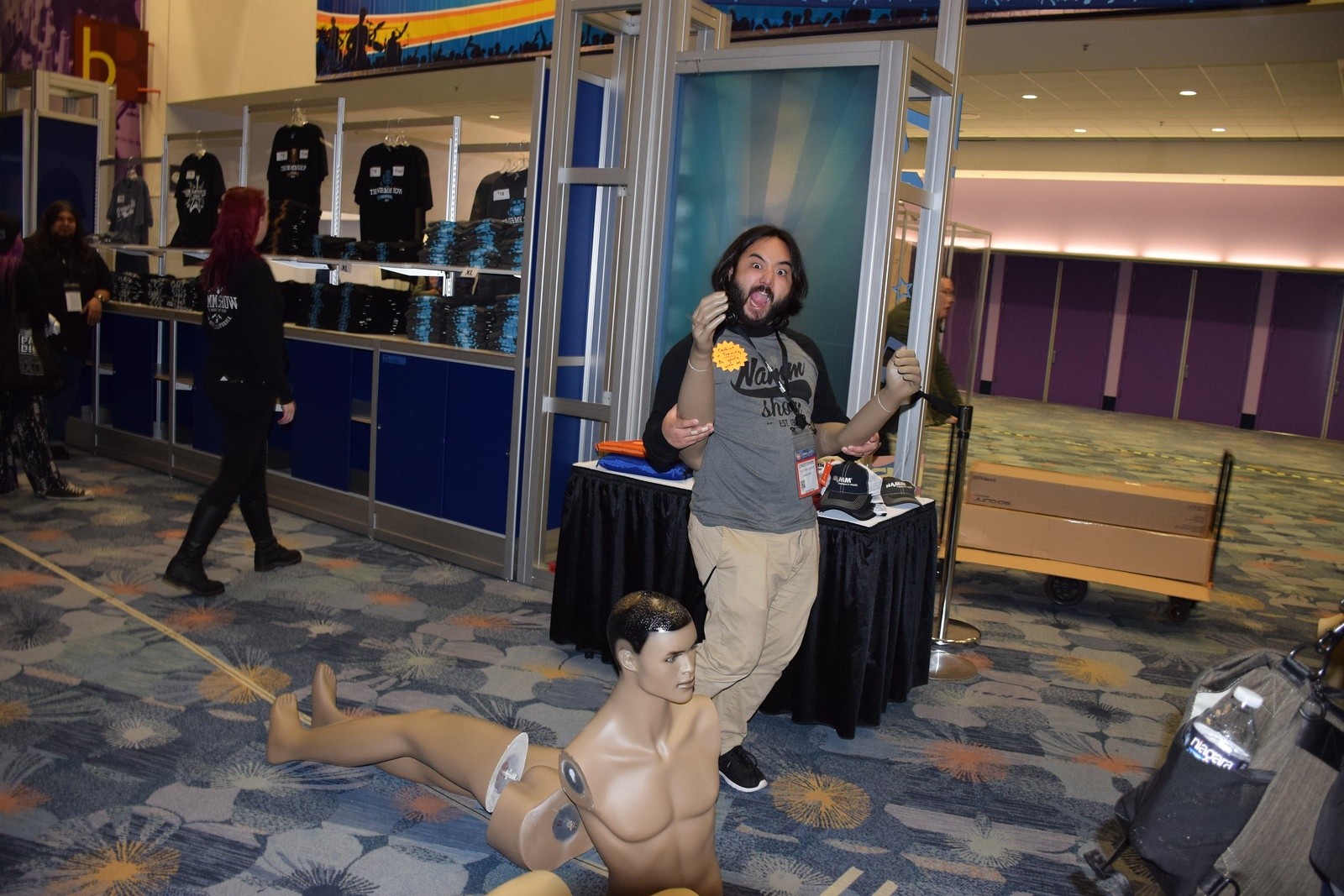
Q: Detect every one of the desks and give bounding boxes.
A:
[547,460,937,740]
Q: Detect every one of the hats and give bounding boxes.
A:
[813,460,925,520]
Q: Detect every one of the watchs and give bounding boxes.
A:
[93,295,105,304]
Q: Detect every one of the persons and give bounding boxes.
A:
[811,345,921,459]
[645,223,881,795]
[0,212,96,501]
[864,273,964,466]
[677,291,728,471]
[161,188,301,596]
[265,590,724,895]
[15,199,114,462]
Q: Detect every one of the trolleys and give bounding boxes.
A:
[936,448,1236,623]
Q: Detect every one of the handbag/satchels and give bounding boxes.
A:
[1072,619,1344,896]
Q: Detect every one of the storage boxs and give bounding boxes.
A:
[956,460,1217,586]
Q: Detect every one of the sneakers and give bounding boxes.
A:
[45,476,95,500]
[718,745,768,793]
[0,483,34,498]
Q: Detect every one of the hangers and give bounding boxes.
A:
[499,142,529,173]
[287,98,309,128]
[383,119,408,147]
[194,130,206,159]
[125,155,137,180]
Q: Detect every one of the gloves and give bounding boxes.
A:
[46,313,61,337]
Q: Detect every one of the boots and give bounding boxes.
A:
[162,500,229,595]
[239,499,302,572]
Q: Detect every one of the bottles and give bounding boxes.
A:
[1183,686,1263,772]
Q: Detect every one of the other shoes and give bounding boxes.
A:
[13,441,21,459]
[50,441,70,461]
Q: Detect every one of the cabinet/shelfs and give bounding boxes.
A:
[0,0,611,583]
